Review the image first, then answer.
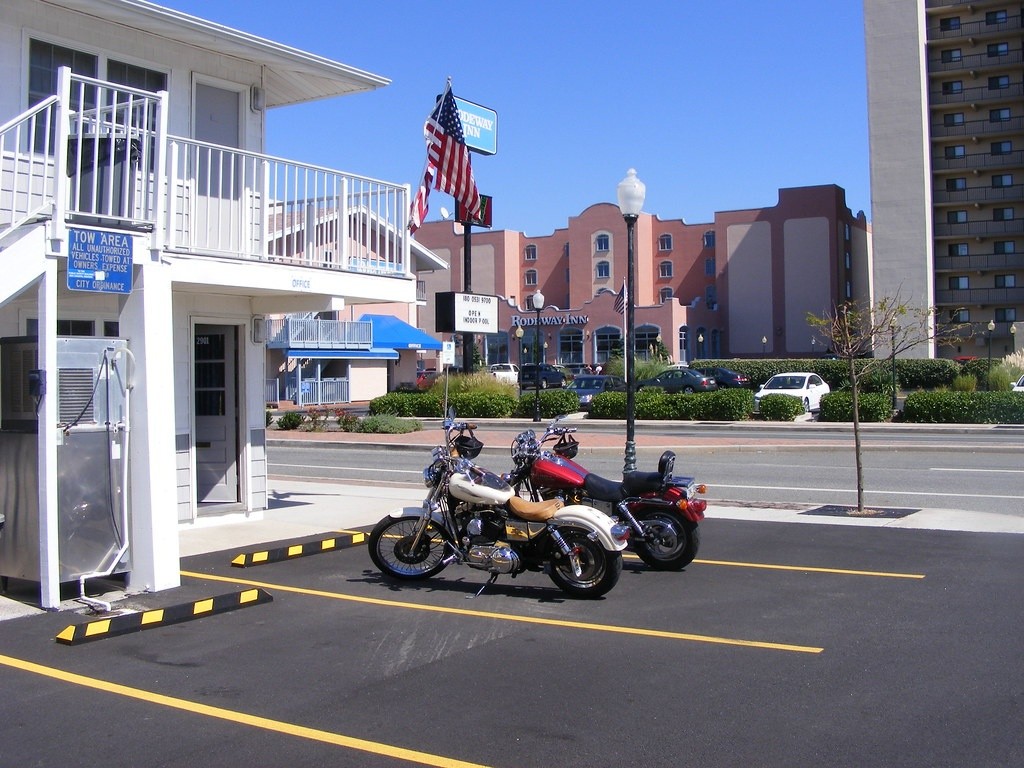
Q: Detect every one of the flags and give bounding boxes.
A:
[412,84,482,232]
[613,287,628,313]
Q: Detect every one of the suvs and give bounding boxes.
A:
[517,363,567,390]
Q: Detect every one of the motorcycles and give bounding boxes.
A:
[367,404,632,603]
[498,416,709,582]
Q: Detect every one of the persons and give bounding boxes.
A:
[595,363,602,374]
[586,363,592,371]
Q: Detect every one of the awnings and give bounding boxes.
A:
[360,315,443,350]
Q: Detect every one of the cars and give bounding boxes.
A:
[636,369,718,394]
[443,365,463,373]
[416,367,436,386]
[696,367,746,389]
[1011,374,1024,392]
[953,355,976,361]
[752,371,831,419]
[565,375,627,407]
[562,362,590,375]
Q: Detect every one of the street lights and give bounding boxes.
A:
[515,318,526,395]
[616,166,648,480]
[655,331,818,360]
[889,312,899,411]
[1009,322,1017,353]
[531,287,545,425]
[986,318,996,393]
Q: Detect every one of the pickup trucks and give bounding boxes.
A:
[489,363,521,384]
